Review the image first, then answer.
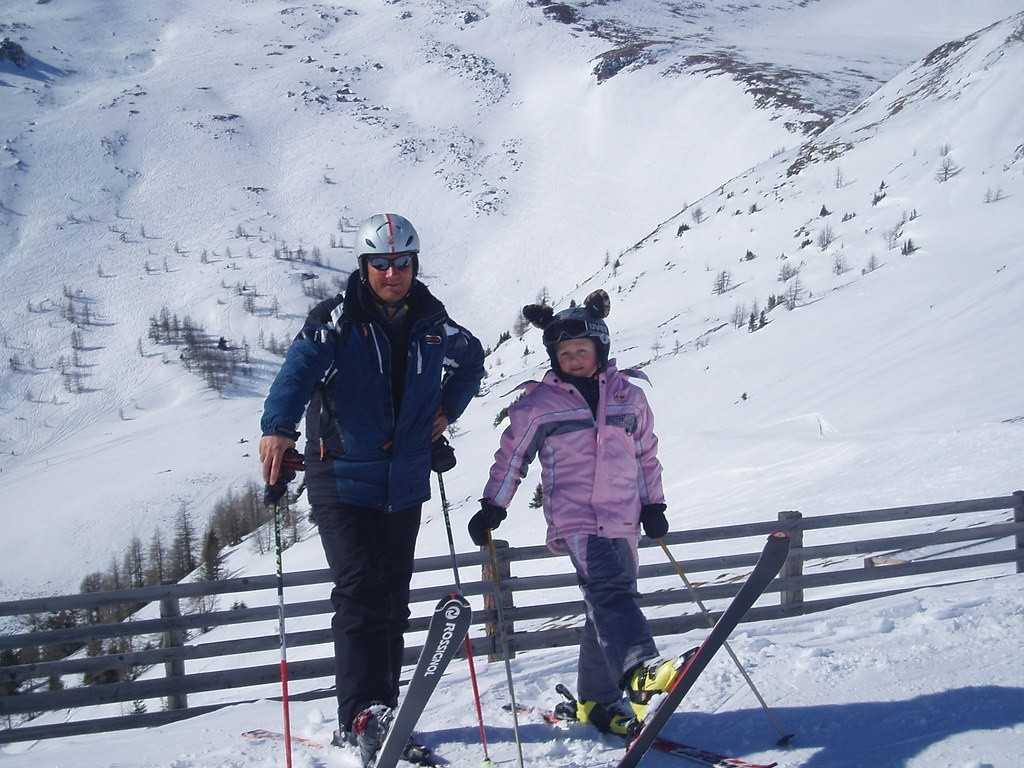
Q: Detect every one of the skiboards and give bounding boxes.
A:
[501,529,792,768]
[237,592,474,768]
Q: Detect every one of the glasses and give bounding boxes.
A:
[362,253,416,271]
[542,318,609,345]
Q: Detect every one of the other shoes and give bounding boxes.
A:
[338,705,433,764]
[574,698,648,735]
[619,647,702,725]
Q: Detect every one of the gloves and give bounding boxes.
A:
[467,505,508,546]
[638,510,668,539]
[262,446,306,509]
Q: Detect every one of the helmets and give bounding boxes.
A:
[522,288,612,360]
[355,213,420,259]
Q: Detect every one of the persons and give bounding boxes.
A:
[260,213,484,768]
[469,287,700,738]
